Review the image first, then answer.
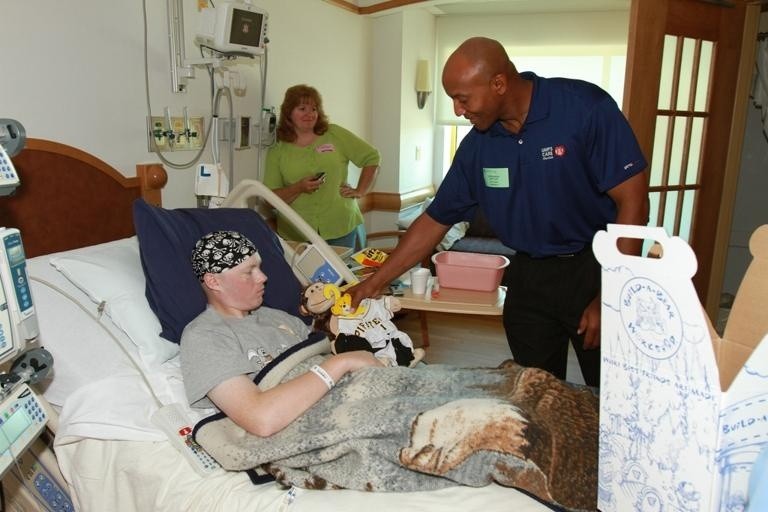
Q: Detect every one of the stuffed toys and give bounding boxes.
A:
[324,284,366,318]
[298,280,425,367]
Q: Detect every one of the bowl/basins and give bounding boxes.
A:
[432,251,510,291]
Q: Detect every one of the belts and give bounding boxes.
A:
[523,248,588,260]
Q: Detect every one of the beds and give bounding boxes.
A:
[0,133,602,511]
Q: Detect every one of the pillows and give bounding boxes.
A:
[132,198,312,346]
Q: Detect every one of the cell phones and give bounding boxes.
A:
[311,171,325,180]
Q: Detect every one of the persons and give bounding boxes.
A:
[180,231,386,437]
[340,37,648,388]
[262,84,379,257]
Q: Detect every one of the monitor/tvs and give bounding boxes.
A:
[229,8,264,47]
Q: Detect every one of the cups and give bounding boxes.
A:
[409,267,429,295]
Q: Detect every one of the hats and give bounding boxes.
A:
[193,231,260,277]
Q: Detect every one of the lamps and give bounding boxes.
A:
[416,58,434,110]
